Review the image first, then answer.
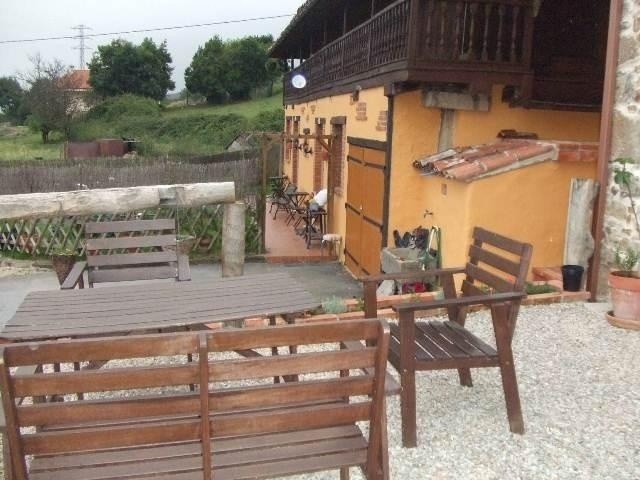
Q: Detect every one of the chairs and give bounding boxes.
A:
[60,219,195,400]
[337,225,533,448]
[268,175,328,248]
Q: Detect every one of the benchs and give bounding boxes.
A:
[0,319,391,479]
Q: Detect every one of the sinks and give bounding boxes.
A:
[378,246,439,285]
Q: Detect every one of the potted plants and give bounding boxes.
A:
[607,156,640,331]
[52,246,77,285]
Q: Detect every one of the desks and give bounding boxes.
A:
[2,272,322,430]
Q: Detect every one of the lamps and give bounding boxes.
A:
[292,138,302,151]
[302,142,313,155]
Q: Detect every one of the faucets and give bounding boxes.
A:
[423,208,434,219]
